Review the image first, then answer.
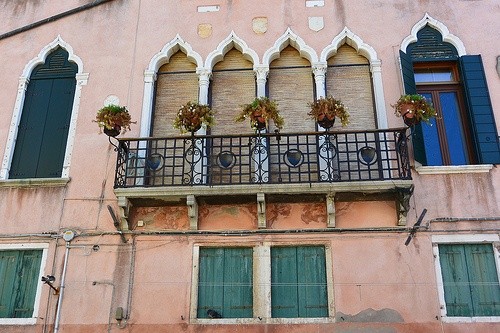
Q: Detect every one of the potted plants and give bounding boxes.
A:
[234,97,285,132]
[93,105,132,137]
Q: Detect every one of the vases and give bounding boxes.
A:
[401,113,422,126]
[183,120,202,133]
[317,116,335,129]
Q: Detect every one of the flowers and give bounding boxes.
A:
[390,93,440,127]
[304,94,351,128]
[173,100,219,133]
